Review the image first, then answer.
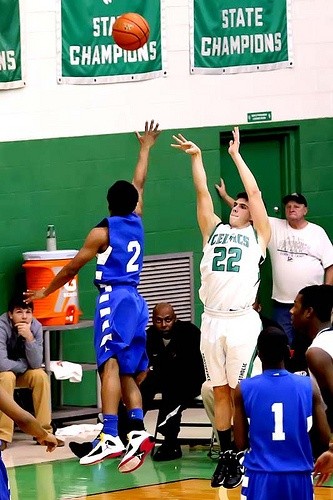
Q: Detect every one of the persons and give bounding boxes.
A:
[0,390,58,500]
[201,294,286,451]
[228,328,333,500]
[22,120,164,475]
[170,126,271,490]
[0,293,65,452]
[288,285,333,408]
[69,302,206,462]
[312,434,333,486]
[214,178,333,369]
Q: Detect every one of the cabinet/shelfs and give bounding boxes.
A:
[38,320,102,434]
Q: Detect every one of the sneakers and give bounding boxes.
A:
[211,450,236,488]
[116,430,156,473]
[78,431,126,466]
[223,449,248,489]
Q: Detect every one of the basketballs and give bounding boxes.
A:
[112,13,150,51]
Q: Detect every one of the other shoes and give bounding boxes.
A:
[37,438,64,446]
[69,442,95,460]
[0,440,7,451]
[152,441,182,461]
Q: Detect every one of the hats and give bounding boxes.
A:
[281,192,306,206]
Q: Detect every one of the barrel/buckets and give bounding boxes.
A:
[22,250,83,326]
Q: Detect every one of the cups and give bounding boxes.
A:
[46,225,56,250]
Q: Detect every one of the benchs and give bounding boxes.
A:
[149,394,216,457]
